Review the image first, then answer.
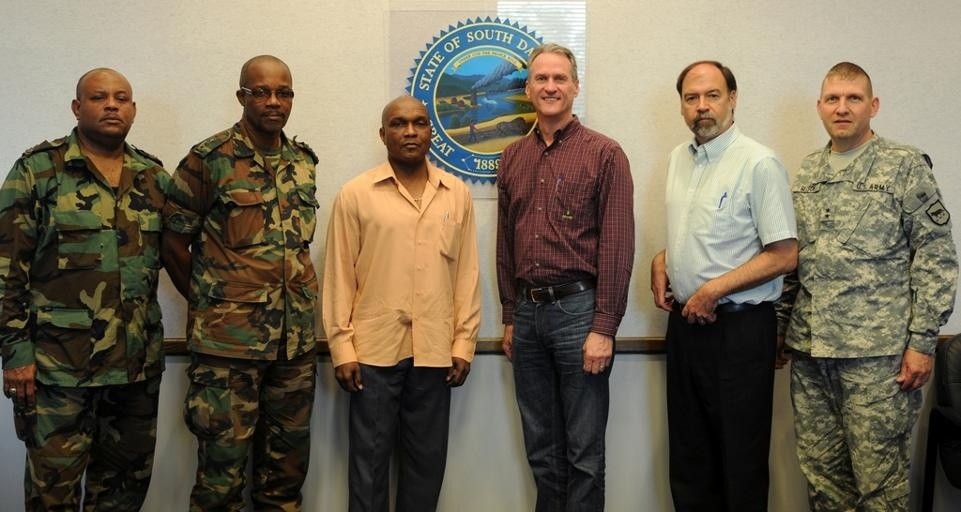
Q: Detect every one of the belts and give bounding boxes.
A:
[517,277,596,303]
[675,297,758,315]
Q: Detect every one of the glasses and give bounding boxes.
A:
[241,85,296,101]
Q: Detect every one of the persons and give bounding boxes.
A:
[775,63,959,512]
[159,55,320,512]
[321,95,481,512]
[652,49,799,512]
[497,44,635,512]
[0,68,171,512]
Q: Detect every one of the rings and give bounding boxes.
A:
[9,388,16,395]
[600,366,605,369]
